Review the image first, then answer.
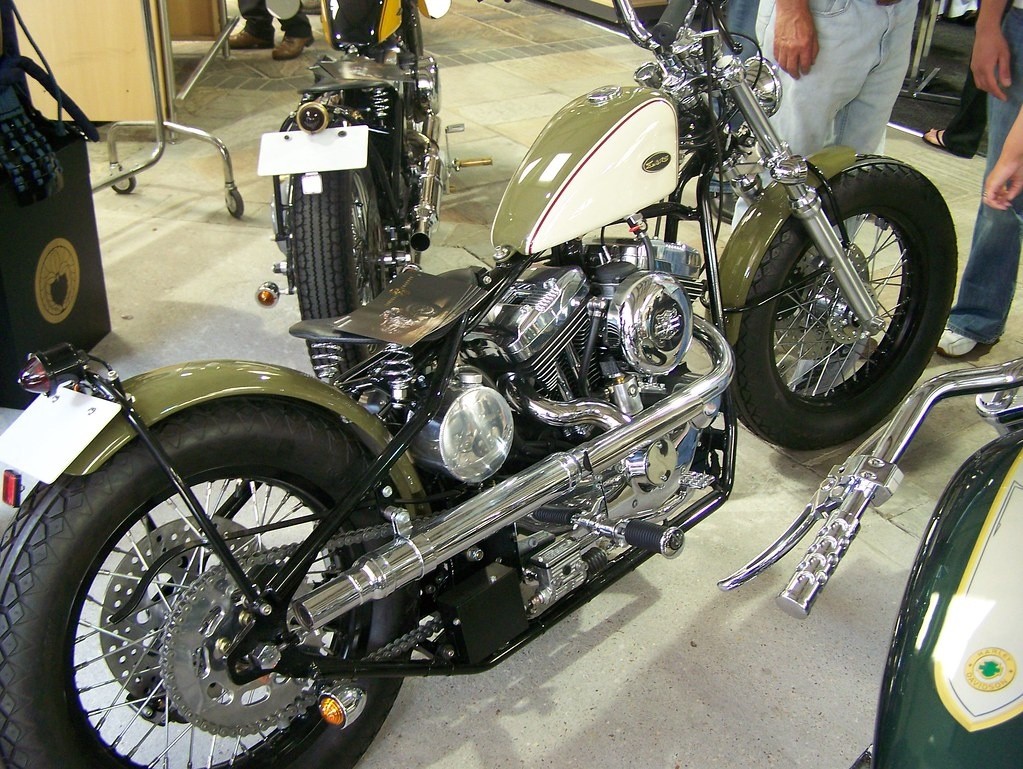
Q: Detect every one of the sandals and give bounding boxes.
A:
[923,129,948,150]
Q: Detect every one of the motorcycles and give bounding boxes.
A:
[714,357,1022,769]
[255,1,462,371]
[0,0,962,767]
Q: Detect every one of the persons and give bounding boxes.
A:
[937,0,1023,357]
[228,0,315,60]
[922,0,1014,160]
[709,0,759,224]
[983,106,1023,211]
[731,0,919,242]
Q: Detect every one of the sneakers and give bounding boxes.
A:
[936,327,978,358]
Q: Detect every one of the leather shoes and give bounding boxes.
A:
[271,35,314,61]
[709,193,739,224]
[228,29,274,50]
